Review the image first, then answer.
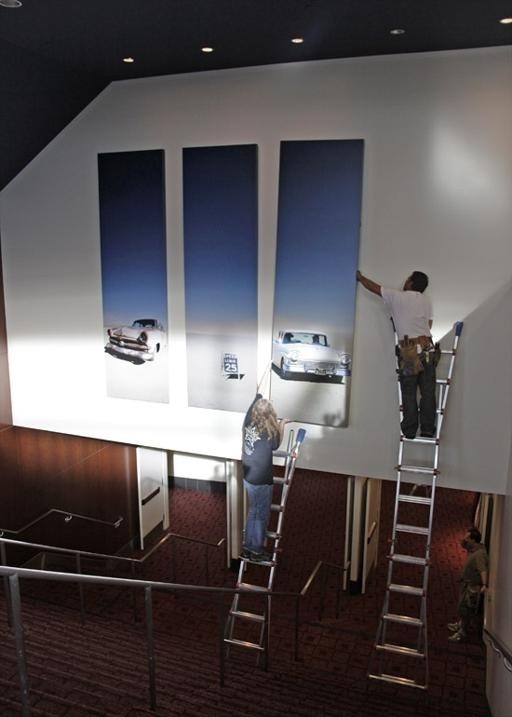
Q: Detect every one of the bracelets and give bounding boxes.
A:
[482,584,487,585]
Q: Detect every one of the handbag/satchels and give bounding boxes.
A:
[400,344,425,378]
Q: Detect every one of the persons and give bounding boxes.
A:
[446,525,489,643]
[357,270,438,439]
[239,391,294,560]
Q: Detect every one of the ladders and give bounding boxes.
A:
[223,428,306,667]
[366,318,462,690]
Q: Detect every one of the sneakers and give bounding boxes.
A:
[447,621,463,643]
[406,426,437,439]
[243,549,271,561]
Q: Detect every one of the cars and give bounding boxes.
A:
[272,328,351,383]
[104,318,168,362]
